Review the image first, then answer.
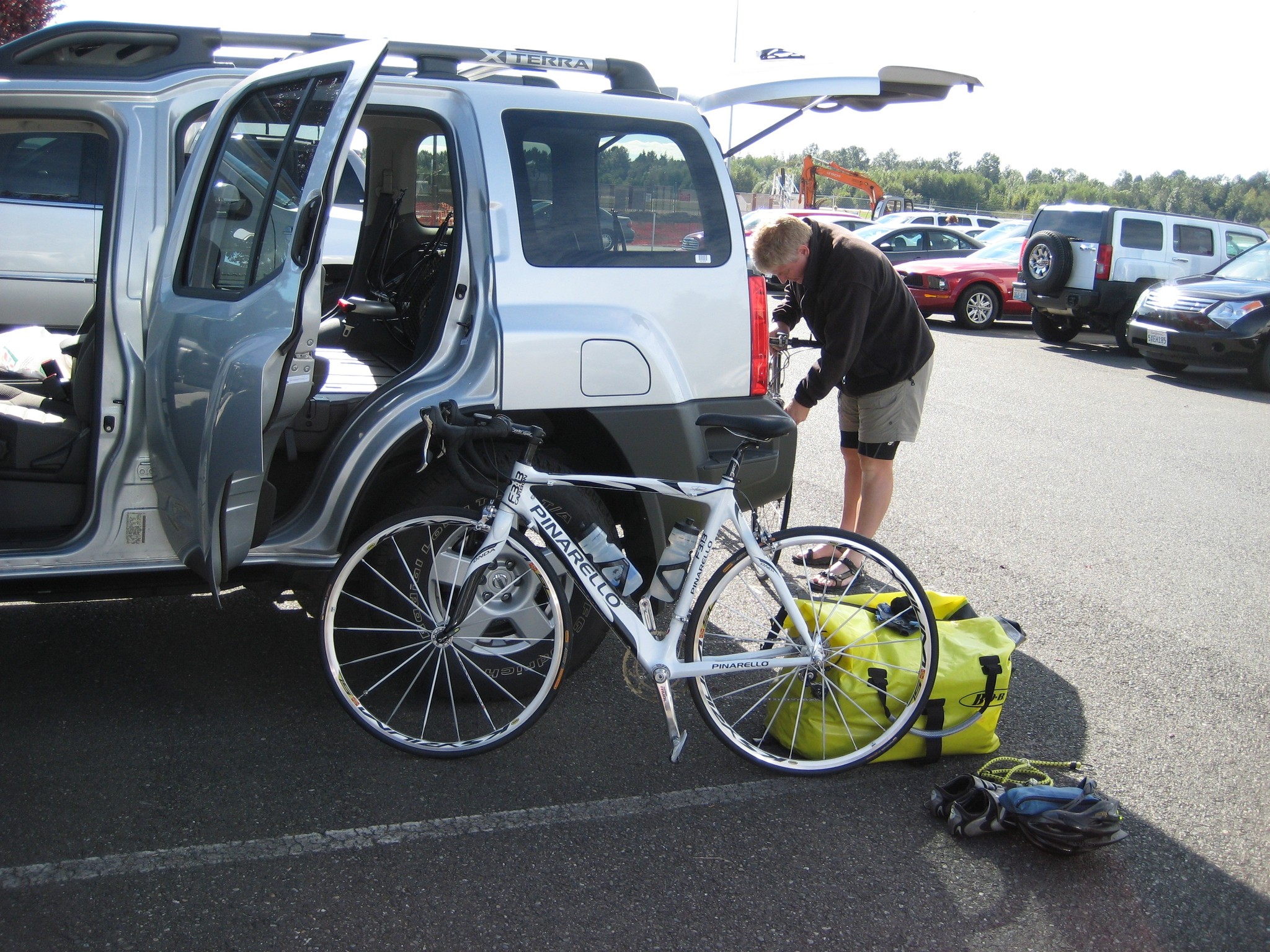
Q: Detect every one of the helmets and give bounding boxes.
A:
[999,777,1126,854]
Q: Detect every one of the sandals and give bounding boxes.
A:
[806,557,867,591]
[793,545,846,563]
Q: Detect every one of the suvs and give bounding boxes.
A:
[1010,203,1270,358]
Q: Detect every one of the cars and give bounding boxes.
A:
[892,235,1033,332]
[678,206,1032,290]
[0,136,365,332]
[533,199,635,251]
[1123,237,1270,392]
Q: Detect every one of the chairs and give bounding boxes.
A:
[0,134,225,535]
[895,237,908,252]
[1175,229,1200,253]
[916,238,921,250]
[937,238,952,250]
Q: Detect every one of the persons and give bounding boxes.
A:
[749,215,935,591]
[945,215,961,226]
[832,205,838,211]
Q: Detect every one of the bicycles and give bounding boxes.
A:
[318,396,936,777]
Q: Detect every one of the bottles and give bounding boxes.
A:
[574,520,643,597]
[650,517,700,602]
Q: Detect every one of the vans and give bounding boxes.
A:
[1,19,988,700]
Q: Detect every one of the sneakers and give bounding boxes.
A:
[948,788,1016,836]
[928,774,1003,818]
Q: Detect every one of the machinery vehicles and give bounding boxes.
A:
[796,149,915,222]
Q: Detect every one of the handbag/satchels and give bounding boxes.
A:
[763,590,1025,763]
